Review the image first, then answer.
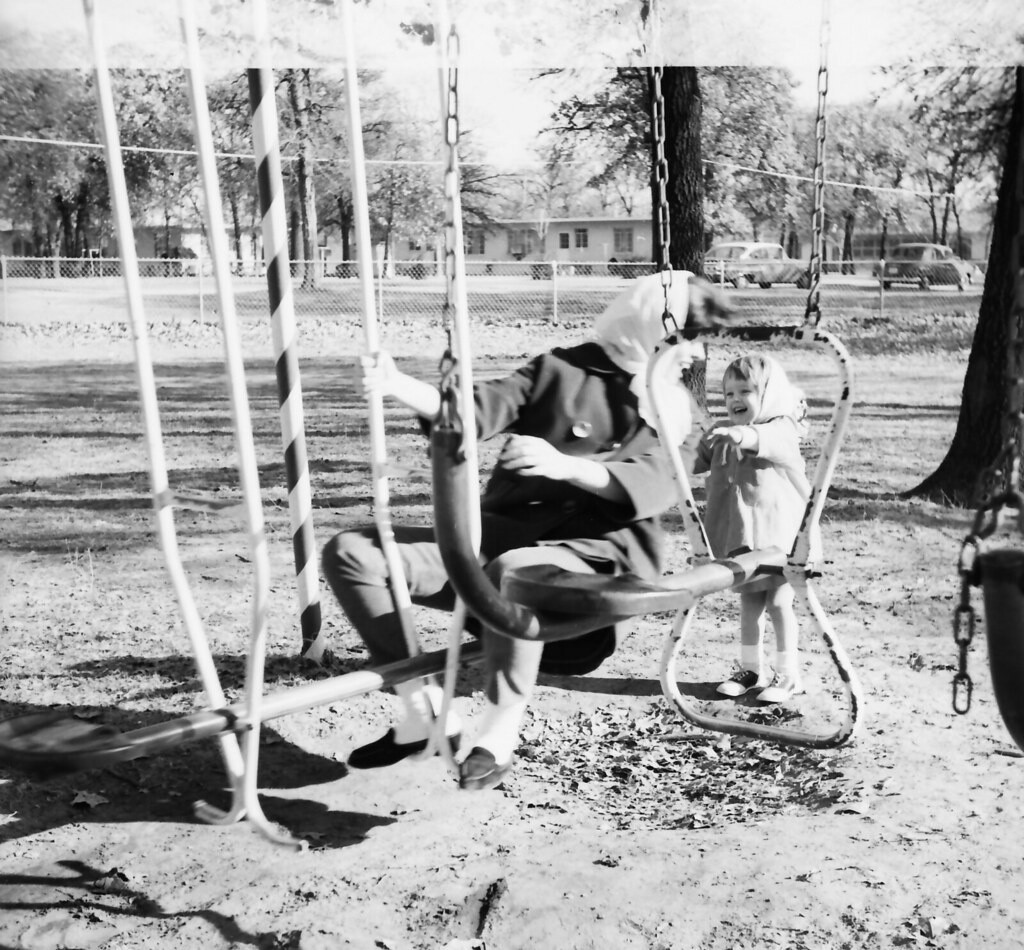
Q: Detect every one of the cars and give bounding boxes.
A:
[879,242,969,293]
[704,241,810,290]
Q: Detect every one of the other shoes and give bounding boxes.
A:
[346,727,457,770]
[755,667,801,702]
[716,659,765,697]
[458,746,515,791]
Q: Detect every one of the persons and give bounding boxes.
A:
[321,271,737,790]
[693,355,812,703]
